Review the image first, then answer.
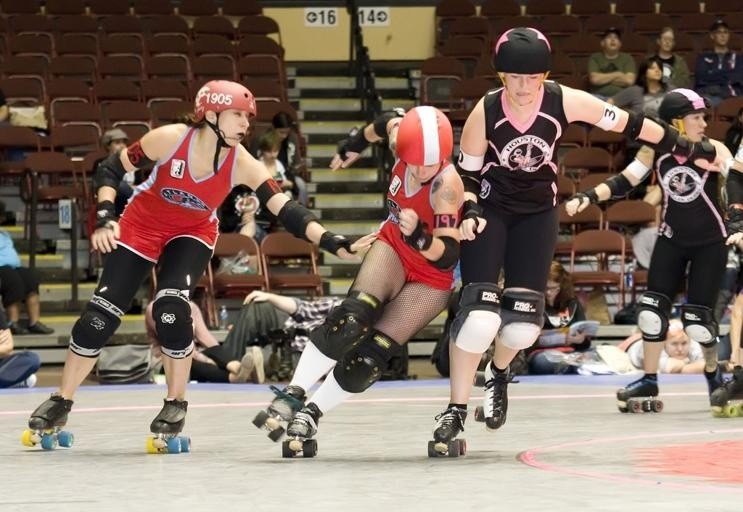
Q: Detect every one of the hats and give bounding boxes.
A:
[597,27,624,39]
[102,129,128,149]
[710,19,728,31]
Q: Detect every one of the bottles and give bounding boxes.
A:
[219,305,229,330]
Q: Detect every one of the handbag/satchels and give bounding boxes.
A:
[7,106,50,129]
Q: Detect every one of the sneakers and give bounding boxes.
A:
[236,354,252,382]
[250,345,266,384]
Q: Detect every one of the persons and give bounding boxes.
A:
[0,299,40,388]
[587,16,742,201]
[526,259,600,373]
[719,141,743,392]
[0,91,10,122]
[267,105,467,437]
[617,319,706,375]
[564,84,742,400]
[0,229,56,336]
[430,23,730,444]
[25,77,379,437]
[91,109,311,278]
[144,283,350,387]
[431,255,528,386]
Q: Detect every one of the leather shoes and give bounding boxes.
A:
[7,321,31,335]
[27,321,54,334]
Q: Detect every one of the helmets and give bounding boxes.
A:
[658,88,710,122]
[493,27,555,74]
[193,80,257,124]
[397,106,454,167]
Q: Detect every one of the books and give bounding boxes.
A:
[537,320,600,350]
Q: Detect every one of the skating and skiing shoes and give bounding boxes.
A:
[428,404,466,457]
[475,359,519,430]
[616,363,743,418]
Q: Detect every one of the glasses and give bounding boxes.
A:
[545,284,562,292]
